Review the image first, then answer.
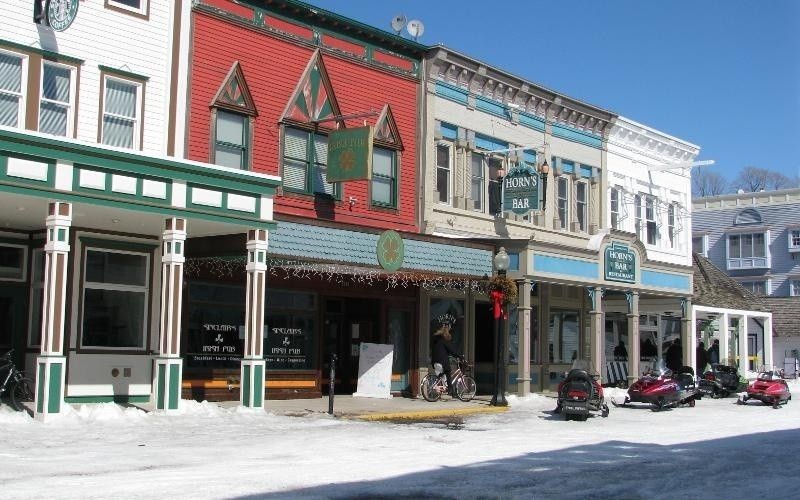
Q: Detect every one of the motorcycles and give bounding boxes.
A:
[554,359,609,421]
[611,359,700,412]
[697,358,741,400]
[738,364,791,409]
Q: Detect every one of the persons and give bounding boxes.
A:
[433,326,464,399]
[613,338,719,378]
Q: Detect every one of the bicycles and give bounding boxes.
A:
[0,348,35,418]
[420,355,477,402]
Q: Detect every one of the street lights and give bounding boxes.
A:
[489,246,510,407]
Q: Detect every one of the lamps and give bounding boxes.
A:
[540,157,549,211]
[496,164,505,212]
